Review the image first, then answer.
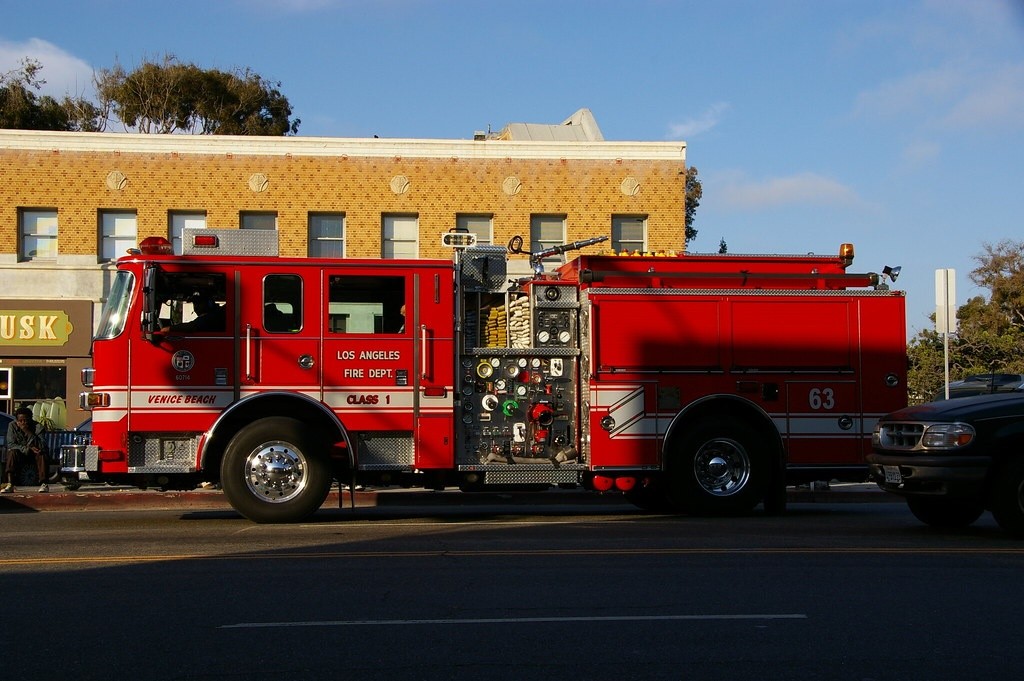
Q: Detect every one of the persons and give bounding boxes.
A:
[397,300,405,334]
[264,298,289,332]
[1,407,49,493]
[160,295,225,332]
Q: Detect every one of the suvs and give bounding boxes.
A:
[870,381,1024,529]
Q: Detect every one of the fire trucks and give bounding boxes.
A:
[81,228,909,522]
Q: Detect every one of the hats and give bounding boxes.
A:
[187,289,214,303]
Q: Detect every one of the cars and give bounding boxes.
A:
[930,375,1023,403]
[1,412,75,484]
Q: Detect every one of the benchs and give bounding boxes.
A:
[41,430,92,482]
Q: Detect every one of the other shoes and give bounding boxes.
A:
[38,484,49,492]
[1,483,13,492]
